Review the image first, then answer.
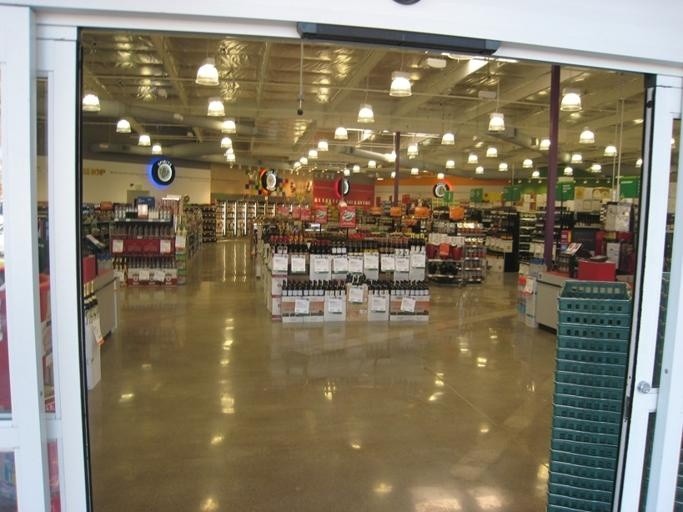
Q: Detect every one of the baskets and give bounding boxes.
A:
[546,277,632,512]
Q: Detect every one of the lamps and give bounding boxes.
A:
[488,113,508,132]
[358,107,375,123]
[195,63,219,85]
[206,99,226,116]
[82,93,100,112]
[559,94,584,111]
[389,77,412,97]
[116,119,643,179]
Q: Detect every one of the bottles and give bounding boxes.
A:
[282,279,346,296]
[366,279,429,296]
[114,206,172,219]
[112,256,177,270]
[346,272,363,285]
[126,224,175,240]
[270,233,425,259]
[276,220,290,234]
[83,279,98,325]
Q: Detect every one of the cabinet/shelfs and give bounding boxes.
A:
[365,197,638,287]
[38,203,216,284]
[211,194,310,237]
